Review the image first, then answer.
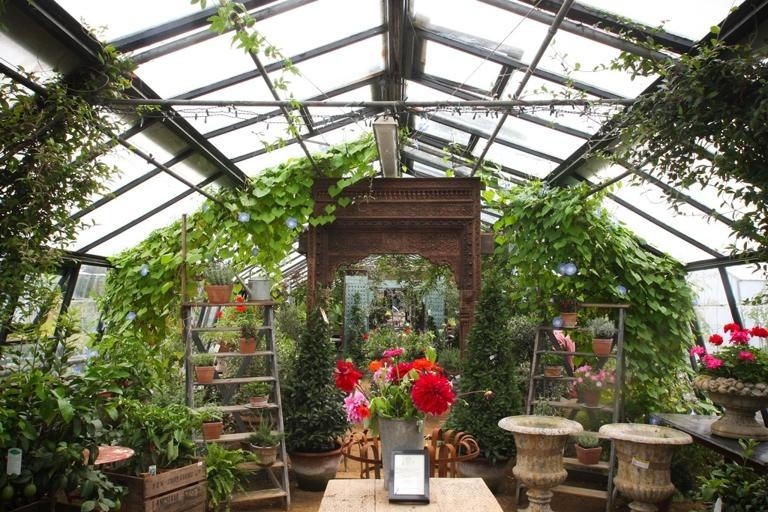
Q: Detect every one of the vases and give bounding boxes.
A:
[378,413,425,490]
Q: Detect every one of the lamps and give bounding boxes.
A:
[370,114,403,180]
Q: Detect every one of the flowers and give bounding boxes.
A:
[285,312,344,491]
[336,348,455,424]
[454,280,514,498]
[695,322,768,443]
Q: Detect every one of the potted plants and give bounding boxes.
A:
[201,404,224,441]
[539,352,564,378]
[557,295,577,329]
[575,430,603,466]
[206,259,233,304]
[244,381,273,408]
[576,366,612,408]
[249,412,282,464]
[237,323,255,356]
[196,355,216,382]
[591,315,616,356]
